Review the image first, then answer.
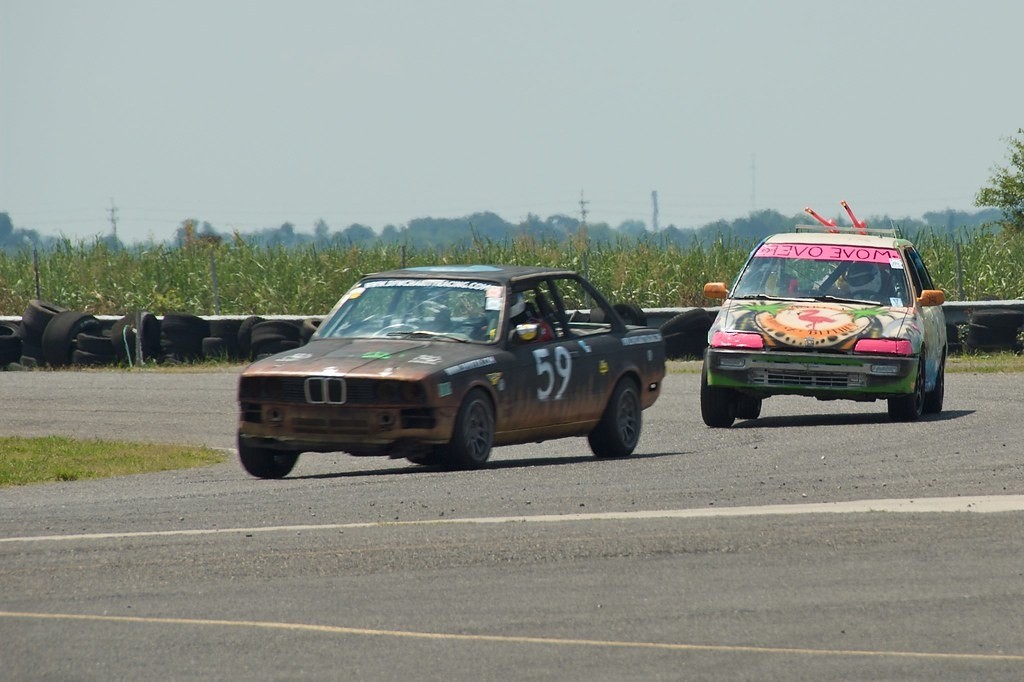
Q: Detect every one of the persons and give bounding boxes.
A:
[840,261,882,300]
[476,291,555,345]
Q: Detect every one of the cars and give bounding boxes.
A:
[700,225,947,426]
[236,263,665,479]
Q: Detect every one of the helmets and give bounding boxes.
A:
[846,262,881,295]
[508,292,525,318]
